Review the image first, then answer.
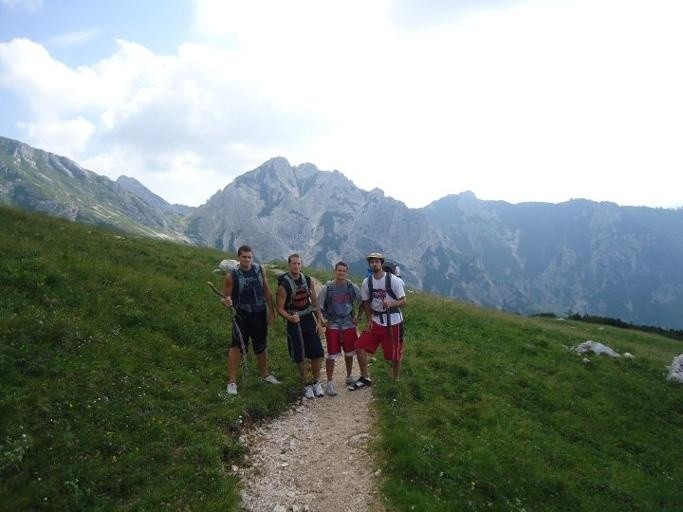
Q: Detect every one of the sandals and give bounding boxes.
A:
[349,376,372,390]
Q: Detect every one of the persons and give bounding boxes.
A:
[219,245,281,397]
[275,253,324,400]
[316,261,364,397]
[346,251,407,392]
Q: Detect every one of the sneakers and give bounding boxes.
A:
[263,375,281,384]
[226,383,238,396]
[298,377,357,400]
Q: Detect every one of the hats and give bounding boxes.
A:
[367,253,384,264]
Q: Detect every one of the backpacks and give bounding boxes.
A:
[369,262,399,315]
[278,272,313,315]
